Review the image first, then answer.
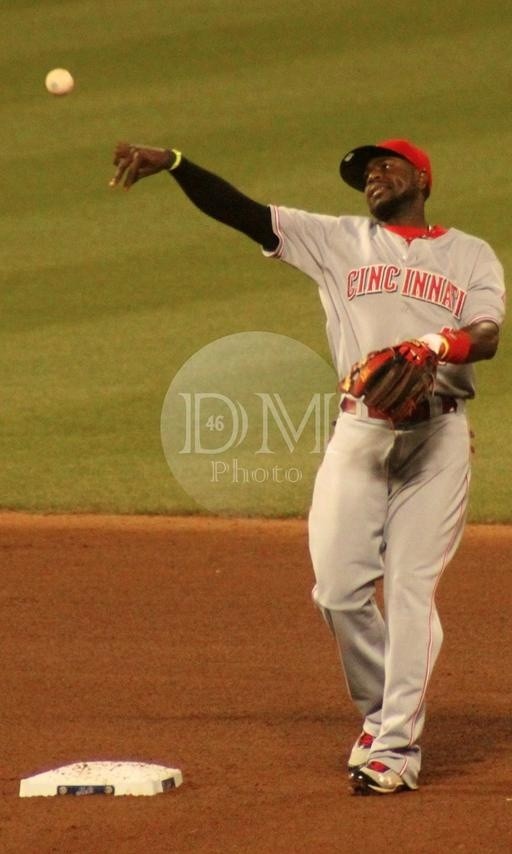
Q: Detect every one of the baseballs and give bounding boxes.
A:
[44,68,74,95]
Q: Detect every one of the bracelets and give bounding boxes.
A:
[168,149,182,172]
[419,326,471,366]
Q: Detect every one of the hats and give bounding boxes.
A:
[340,142,430,192]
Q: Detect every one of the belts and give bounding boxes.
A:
[341,397,456,423]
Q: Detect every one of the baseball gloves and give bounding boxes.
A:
[340,342,440,427]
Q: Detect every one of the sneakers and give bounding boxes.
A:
[346,729,421,795]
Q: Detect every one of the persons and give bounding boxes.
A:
[109,137,507,795]
